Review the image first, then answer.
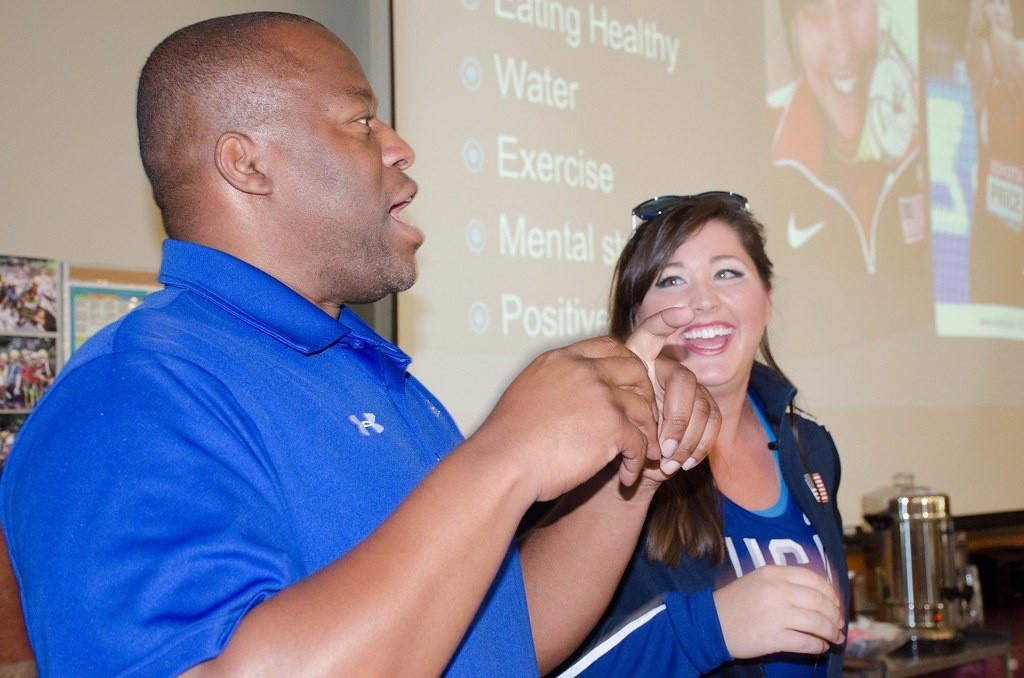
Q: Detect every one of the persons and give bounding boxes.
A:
[0,255,56,462]
[762,0,923,350]
[964,0,1024,308]
[543,191,856,678]
[0,11,724,678]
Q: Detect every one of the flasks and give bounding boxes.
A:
[891,495,956,627]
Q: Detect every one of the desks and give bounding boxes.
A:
[844,625,1016,678]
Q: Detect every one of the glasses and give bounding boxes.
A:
[631,192,750,241]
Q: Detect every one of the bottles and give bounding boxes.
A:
[862,472,965,656]
[845,527,873,616]
[954,533,985,632]
